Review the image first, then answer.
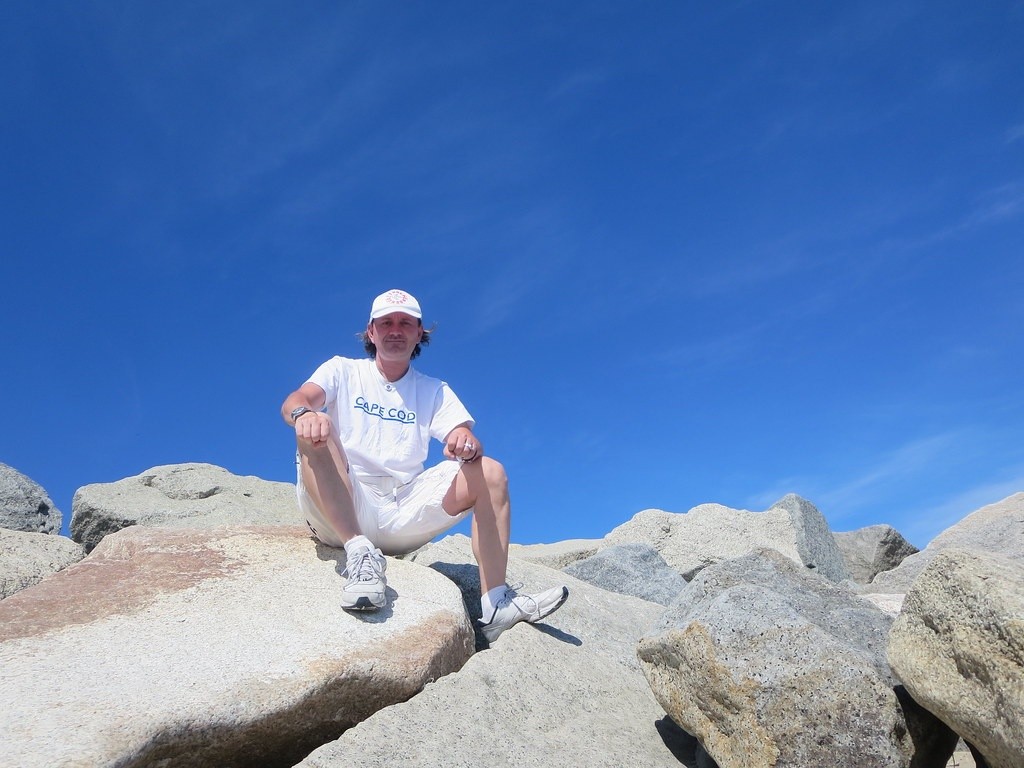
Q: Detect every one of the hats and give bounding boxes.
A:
[369,289,422,323]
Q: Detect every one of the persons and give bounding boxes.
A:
[281,289,570,644]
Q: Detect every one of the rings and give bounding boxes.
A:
[464,443,474,450]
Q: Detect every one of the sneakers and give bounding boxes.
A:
[477,582,568,648]
[341,546,387,610]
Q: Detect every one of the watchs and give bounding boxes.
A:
[290,406,313,424]
